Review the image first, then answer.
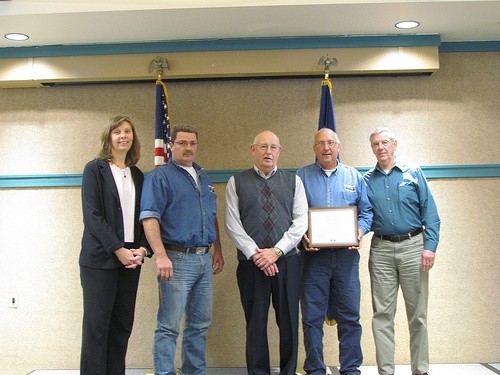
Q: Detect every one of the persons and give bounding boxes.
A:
[139,125,224,375]
[297,128,373,375]
[362,127,440,375]
[78,116,154,375]
[224,130,310,375]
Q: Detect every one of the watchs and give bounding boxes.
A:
[274,247,283,256]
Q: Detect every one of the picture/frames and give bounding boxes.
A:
[307,206,359,248]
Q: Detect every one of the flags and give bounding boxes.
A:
[316,80,341,326]
[154,81,172,168]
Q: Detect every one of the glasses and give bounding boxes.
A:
[172,140,199,146]
[254,144,279,151]
[315,141,340,147]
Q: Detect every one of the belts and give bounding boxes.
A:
[163,243,212,255]
[374,227,424,242]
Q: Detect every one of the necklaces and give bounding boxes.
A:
[114,163,127,178]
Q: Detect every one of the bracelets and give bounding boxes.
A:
[140,246,149,256]
